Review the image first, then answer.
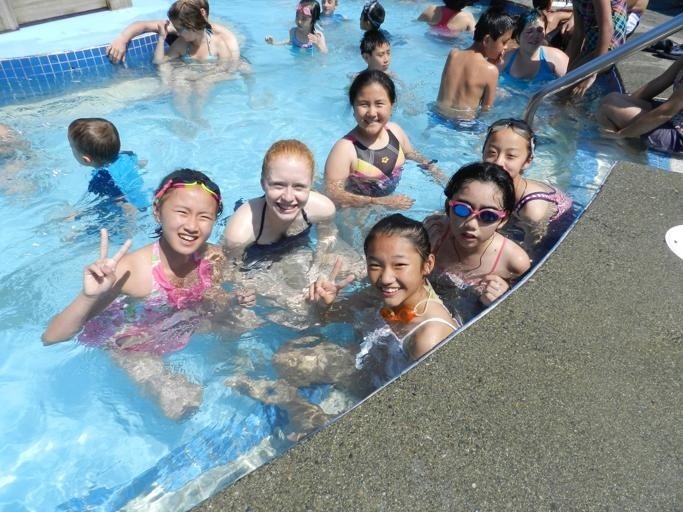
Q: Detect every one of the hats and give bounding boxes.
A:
[362,0,387,29]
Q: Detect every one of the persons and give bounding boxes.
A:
[0,124,39,196]
[326,70,446,224]
[268,162,530,330]
[106,0,258,110]
[266,0,396,72]
[152,0,233,116]
[226,140,362,329]
[482,117,572,257]
[224,215,458,443]
[423,0,683,156]
[40,168,266,419]
[58,118,151,244]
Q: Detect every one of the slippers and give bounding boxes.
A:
[657,43,683,62]
[641,39,674,52]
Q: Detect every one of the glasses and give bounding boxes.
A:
[296,3,313,19]
[447,199,508,224]
[488,115,535,155]
[156,174,222,200]
[376,304,415,324]
